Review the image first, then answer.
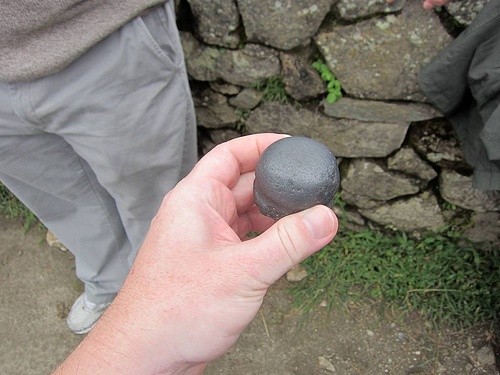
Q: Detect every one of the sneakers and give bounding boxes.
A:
[67,292,111,335]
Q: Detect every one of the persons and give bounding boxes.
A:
[0,0,198,335]
[52,132,338,375]
